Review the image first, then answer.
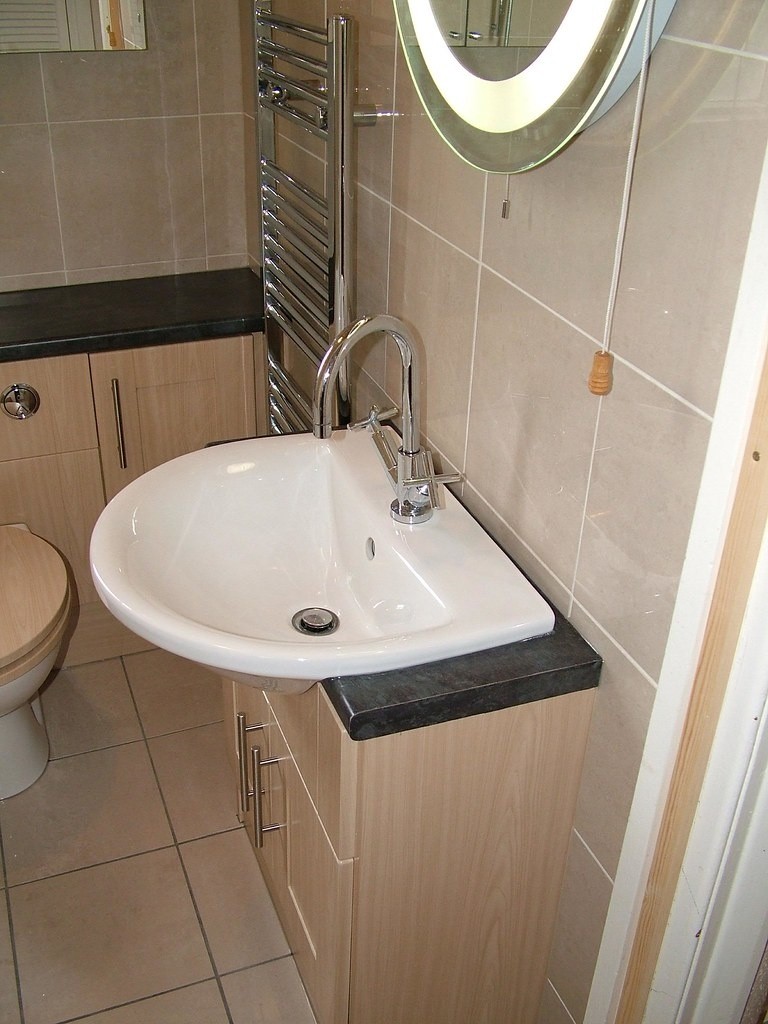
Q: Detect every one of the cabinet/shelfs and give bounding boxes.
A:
[0,265,288,668]
[204,422,602,1024]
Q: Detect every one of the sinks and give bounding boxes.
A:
[89,424,557,695]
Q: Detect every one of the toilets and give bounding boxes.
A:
[1,521,75,802]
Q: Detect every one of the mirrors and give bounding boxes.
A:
[0,0,149,54]
[392,0,679,177]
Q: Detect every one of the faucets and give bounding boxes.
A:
[312,311,468,526]
